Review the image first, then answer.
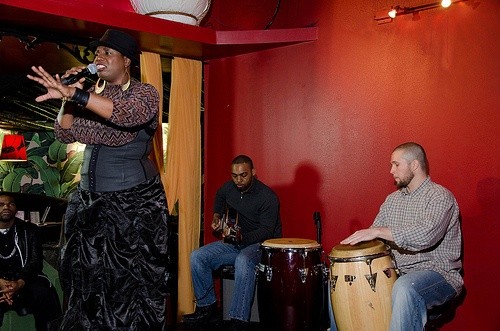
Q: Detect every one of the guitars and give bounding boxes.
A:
[211,206,241,243]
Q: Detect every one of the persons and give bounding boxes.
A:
[327,142,464,331]
[26,26,169,331]
[0,191,63,331]
[183,155,282,331]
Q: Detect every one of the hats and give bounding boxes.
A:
[90,28,140,62]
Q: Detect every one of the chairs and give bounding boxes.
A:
[0,260,63,331]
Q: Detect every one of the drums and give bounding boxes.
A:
[328,239,399,331]
[256,238,323,331]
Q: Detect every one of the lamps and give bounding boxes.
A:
[388,5,404,19]
[0,126,28,162]
[441,0,451,8]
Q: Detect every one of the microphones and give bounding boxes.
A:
[60,64,98,86]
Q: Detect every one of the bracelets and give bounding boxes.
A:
[71,86,90,108]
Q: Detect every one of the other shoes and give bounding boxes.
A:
[183,306,217,323]
[229,318,248,331]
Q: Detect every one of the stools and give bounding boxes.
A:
[216,265,257,320]
[424,300,451,331]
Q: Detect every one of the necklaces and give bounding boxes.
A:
[240,191,243,200]
